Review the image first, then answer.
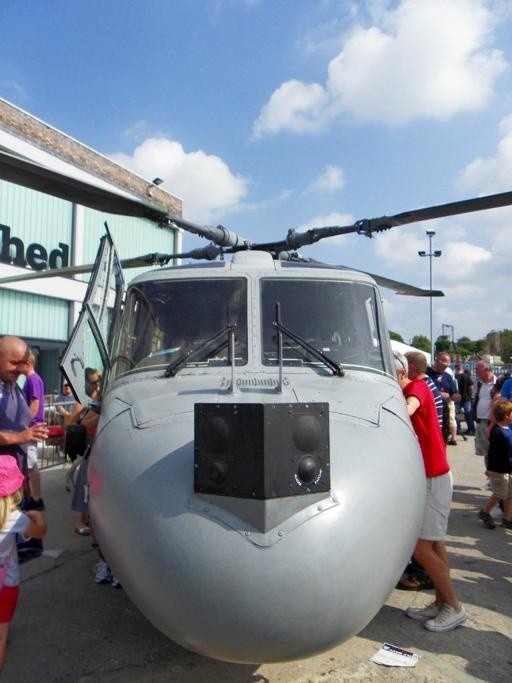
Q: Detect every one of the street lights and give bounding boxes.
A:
[415,225,445,366]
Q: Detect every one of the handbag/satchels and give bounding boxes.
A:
[63,422,88,457]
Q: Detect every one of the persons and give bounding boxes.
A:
[0,335,49,669]
[392,348,512,634]
[54,367,104,536]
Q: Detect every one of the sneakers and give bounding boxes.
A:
[500,519,512,528]
[424,602,468,633]
[22,496,44,511]
[407,600,441,622]
[478,508,496,530]
[94,558,121,587]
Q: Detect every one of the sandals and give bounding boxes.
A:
[398,572,435,591]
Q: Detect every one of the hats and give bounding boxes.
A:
[0,454,25,499]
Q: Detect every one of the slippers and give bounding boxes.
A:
[75,525,92,536]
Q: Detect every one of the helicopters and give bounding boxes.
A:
[0,148,512,671]
